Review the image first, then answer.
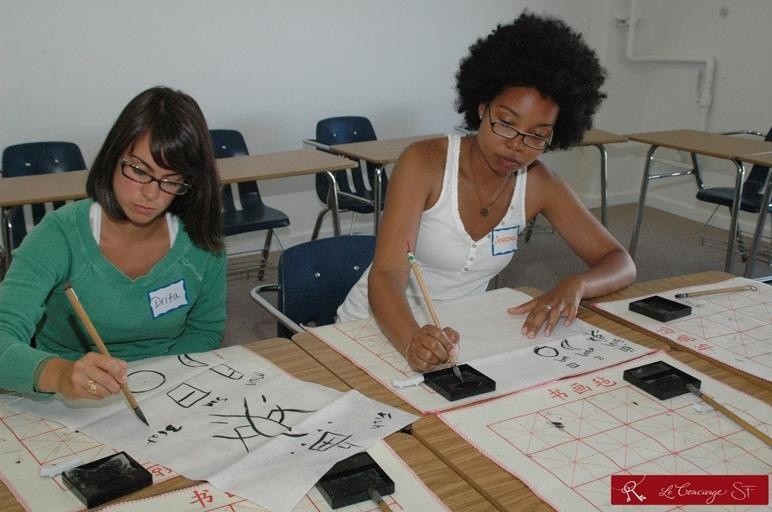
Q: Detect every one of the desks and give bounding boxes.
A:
[0,150,358,236]
[623,129,772,273]
[330,127,629,235]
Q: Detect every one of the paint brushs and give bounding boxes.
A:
[61,282,151,427]
[405,241,464,383]
[367,486,392,512]
[685,383,772,448]
[675,286,758,298]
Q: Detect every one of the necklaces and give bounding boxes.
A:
[469,149,510,218]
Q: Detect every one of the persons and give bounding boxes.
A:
[1,86,227,401]
[336,13,637,372]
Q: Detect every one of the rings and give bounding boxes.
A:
[85,382,97,396]
[540,304,554,311]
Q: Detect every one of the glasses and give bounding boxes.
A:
[117,152,197,197]
[484,103,555,152]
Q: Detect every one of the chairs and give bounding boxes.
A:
[209,129,290,281]
[249,235,375,339]
[0,142,88,270]
[692,127,772,263]
[303,116,389,240]
